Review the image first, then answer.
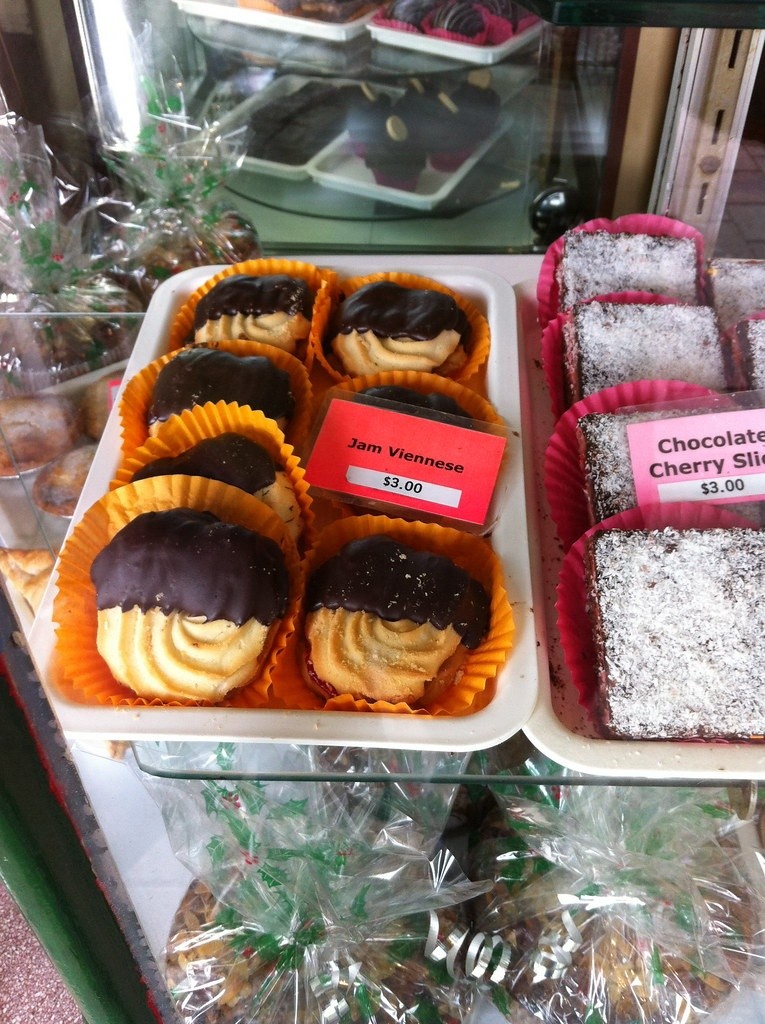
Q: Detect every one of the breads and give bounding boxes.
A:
[153,747,765,1023]
[0,212,258,621]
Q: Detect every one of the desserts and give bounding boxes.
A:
[51,258,519,720]
[232,0,545,195]
[535,210,765,742]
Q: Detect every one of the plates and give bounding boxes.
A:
[28,255,541,754]
[365,18,554,66]
[307,89,515,211]
[492,64,541,110]
[508,256,765,780]
[194,72,390,182]
[172,0,383,43]
